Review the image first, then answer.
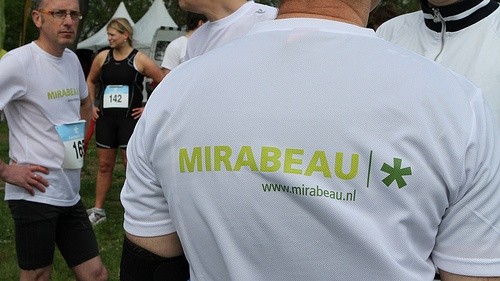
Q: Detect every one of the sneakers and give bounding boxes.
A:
[86,207,106,226]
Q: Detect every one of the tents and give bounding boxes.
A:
[127,0,177,60]
[77,1,136,53]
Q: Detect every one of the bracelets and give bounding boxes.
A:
[88,102,94,110]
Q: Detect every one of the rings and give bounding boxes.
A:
[32,174,37,180]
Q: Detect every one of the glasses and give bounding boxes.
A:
[36,9,83,20]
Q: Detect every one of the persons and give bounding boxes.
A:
[119,0,278,281]
[120,1,499,281]
[85,17,165,227]
[159,11,210,78]
[0,1,112,281]
[374,0,500,111]
